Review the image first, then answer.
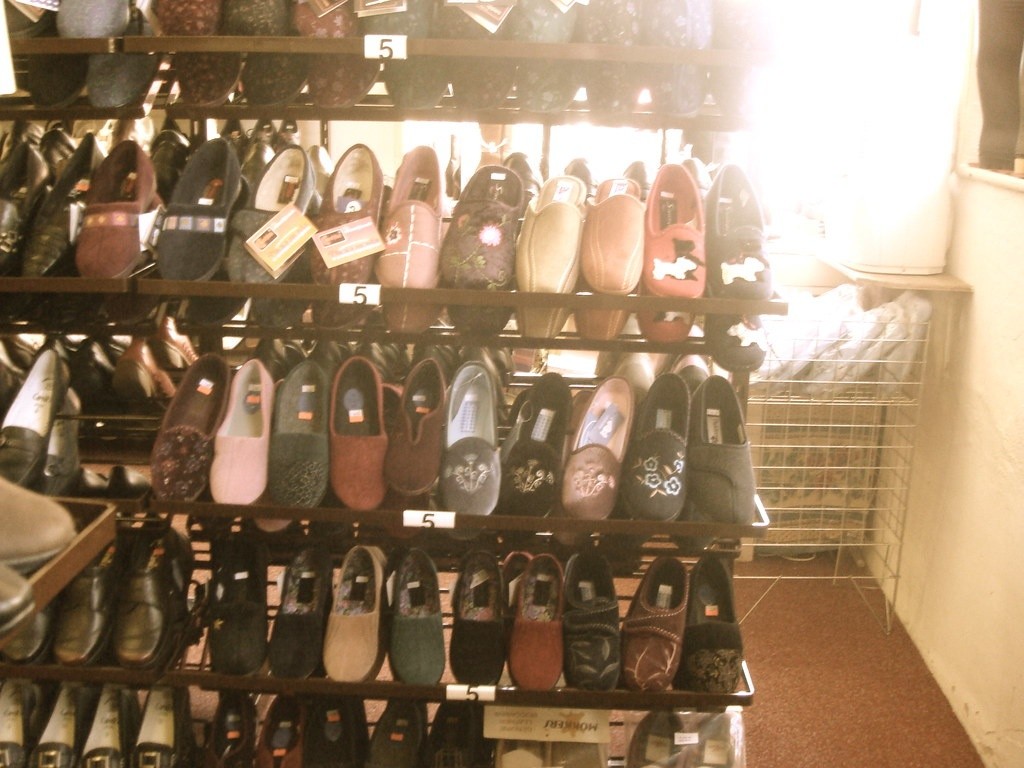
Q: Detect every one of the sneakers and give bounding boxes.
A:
[3,1,757,125]
[0,478,746,767]
[0,113,776,301]
[1,282,758,534]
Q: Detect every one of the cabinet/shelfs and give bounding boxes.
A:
[0,0,969,768]
